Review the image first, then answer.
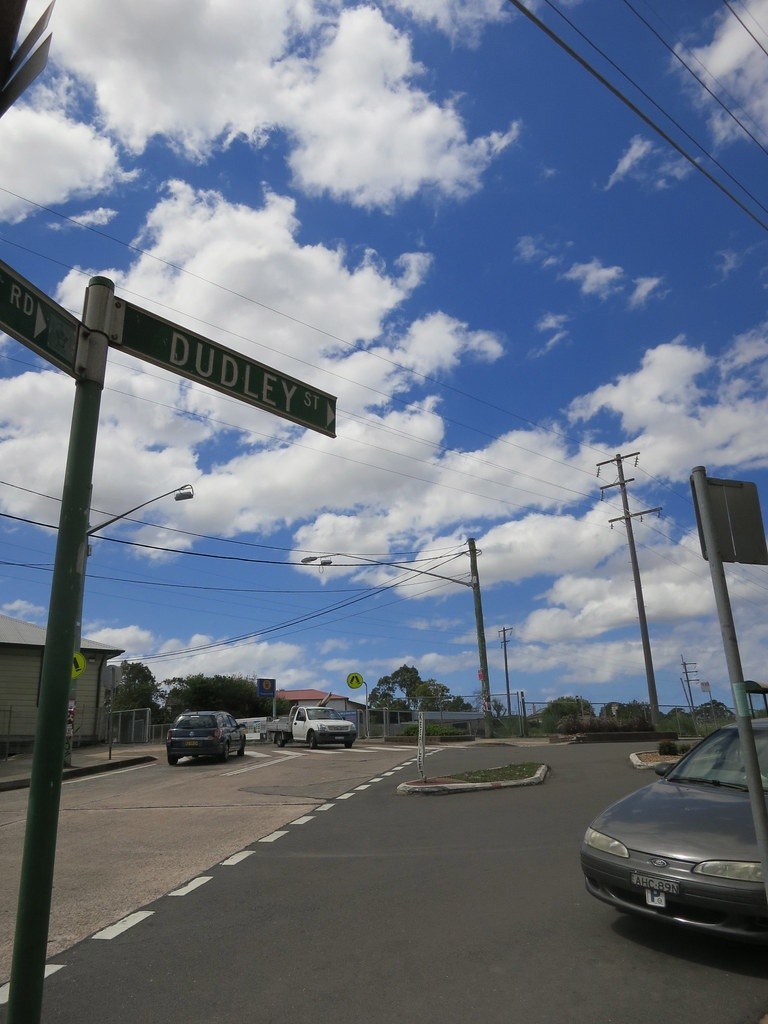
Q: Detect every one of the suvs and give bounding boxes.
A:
[165,709,247,765]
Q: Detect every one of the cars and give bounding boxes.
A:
[579,718,768,942]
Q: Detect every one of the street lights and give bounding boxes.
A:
[61,482,194,770]
[301,552,493,740]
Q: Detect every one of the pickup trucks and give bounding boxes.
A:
[264,706,357,749]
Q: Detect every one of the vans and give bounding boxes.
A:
[235,717,271,742]
